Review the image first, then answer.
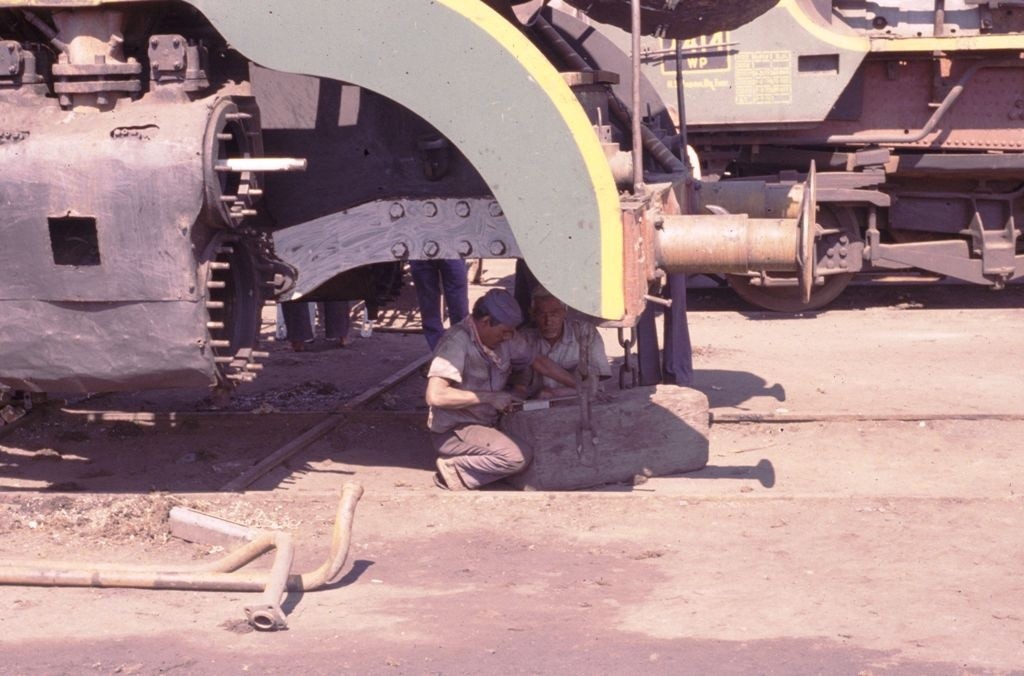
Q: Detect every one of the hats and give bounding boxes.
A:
[475,288,523,328]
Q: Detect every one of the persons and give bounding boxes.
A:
[512,279,613,411]
[275,258,468,352]
[425,288,617,491]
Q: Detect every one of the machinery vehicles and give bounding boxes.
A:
[0,0,1024,431]
